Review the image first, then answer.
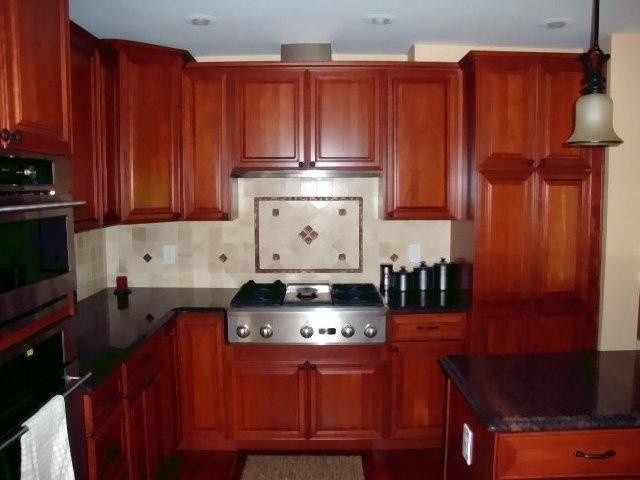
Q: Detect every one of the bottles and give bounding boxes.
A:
[397,291,447,309]
[396,266,413,292]
[434,256,454,291]
[413,261,434,291]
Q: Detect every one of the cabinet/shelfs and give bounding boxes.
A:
[230,61,383,172]
[225,343,388,442]
[100,38,196,228]
[380,60,462,221]
[83,362,128,480]
[0,0,75,162]
[389,307,469,452]
[183,61,239,222]
[450,49,609,357]
[175,308,236,452]
[123,325,184,480]
[68,18,102,234]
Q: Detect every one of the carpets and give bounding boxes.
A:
[231,448,374,480]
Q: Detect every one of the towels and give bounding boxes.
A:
[19,394,76,480]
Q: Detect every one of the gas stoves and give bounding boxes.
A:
[226,278,387,346]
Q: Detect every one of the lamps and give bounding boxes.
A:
[563,0,624,148]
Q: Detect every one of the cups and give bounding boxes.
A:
[116,275,128,293]
[380,263,393,291]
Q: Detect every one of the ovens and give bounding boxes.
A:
[0,149,93,480]
